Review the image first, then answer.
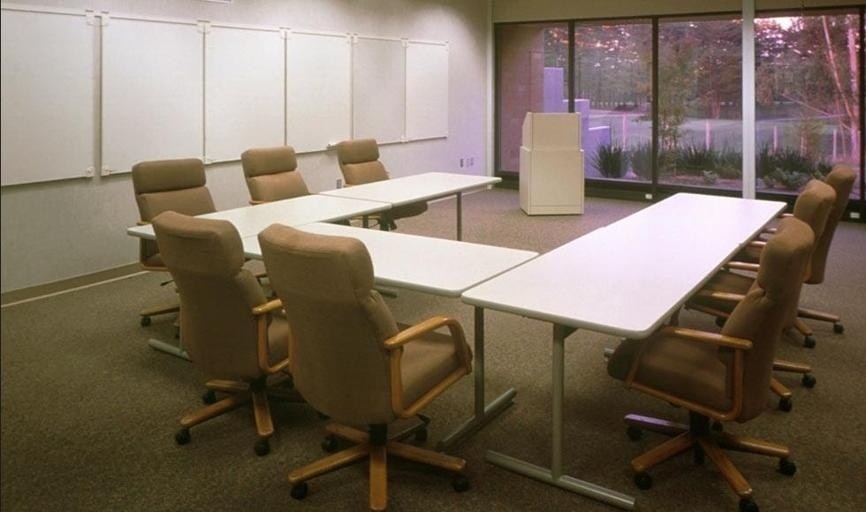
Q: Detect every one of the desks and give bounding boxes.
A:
[238,220,538,454]
[127,194,394,259]
[460,193,786,505]
[323,169,503,298]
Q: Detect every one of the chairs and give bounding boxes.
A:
[716,165,856,348]
[257,222,474,509]
[128,157,252,341]
[609,218,811,502]
[240,144,348,278]
[151,210,296,455]
[334,137,428,231]
[664,179,837,408]
